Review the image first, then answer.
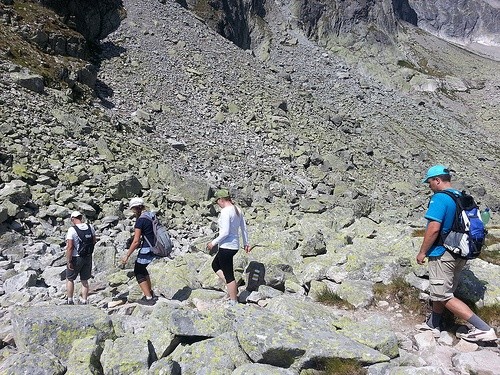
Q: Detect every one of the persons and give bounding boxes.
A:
[121,198,157,305]
[416,165,498,342]
[206,190,251,308]
[58,211,97,305]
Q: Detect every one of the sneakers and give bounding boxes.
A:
[461,326,497,341]
[415,322,440,338]
[138,296,156,306]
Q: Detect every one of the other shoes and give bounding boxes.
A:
[79,300,86,305]
[65,301,74,305]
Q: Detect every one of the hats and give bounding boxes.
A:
[214,190,230,202]
[129,198,144,209]
[70,211,83,219]
[422,166,450,184]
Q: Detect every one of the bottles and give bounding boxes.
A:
[480,208,490,226]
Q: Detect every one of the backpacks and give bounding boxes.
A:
[139,215,172,257]
[436,190,487,260]
[73,225,94,257]
